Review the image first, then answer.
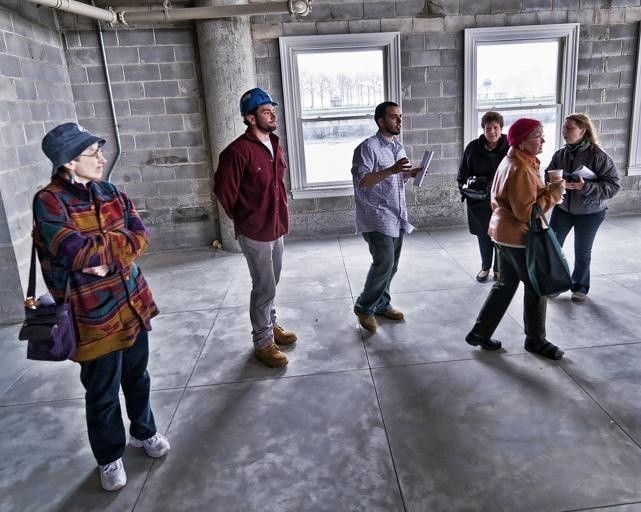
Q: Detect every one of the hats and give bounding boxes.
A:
[42,122,107,176]
[506,118,540,146]
[240,88,279,116]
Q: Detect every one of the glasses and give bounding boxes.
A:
[78,148,104,159]
[529,134,545,139]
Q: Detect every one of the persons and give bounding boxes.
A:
[351,103,431,332]
[209,87,297,370]
[33,122,169,493]
[544,112,623,304]
[456,113,510,281]
[464,119,566,359]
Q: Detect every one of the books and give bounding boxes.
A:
[570,165,599,180]
[414,149,435,188]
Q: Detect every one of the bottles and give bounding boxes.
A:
[24,296,37,309]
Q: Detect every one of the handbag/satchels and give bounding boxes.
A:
[461,175,492,198]
[525,203,572,296]
[18,300,77,362]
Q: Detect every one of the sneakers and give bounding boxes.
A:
[273,323,297,344]
[476,269,489,281]
[571,292,585,302]
[255,343,289,368]
[376,307,404,320]
[465,331,501,350]
[524,340,562,360]
[100,457,128,491]
[354,311,377,333]
[129,433,171,458]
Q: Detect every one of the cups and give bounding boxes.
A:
[548,170,565,185]
